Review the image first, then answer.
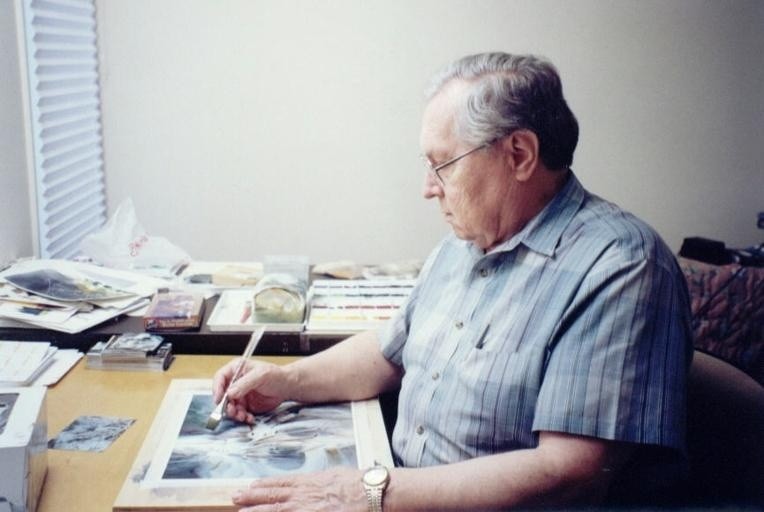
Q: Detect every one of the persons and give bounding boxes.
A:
[213,50,692,512]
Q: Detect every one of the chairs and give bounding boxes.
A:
[687,348,764,511]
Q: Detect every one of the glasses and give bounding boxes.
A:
[419,140,494,187]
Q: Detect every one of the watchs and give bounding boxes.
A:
[361,462,390,512]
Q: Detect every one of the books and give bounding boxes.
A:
[99,333,147,360]
[84,341,176,372]
[110,332,164,353]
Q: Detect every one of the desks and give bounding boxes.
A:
[0,260,422,512]
[673,254,763,374]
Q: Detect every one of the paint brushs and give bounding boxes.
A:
[206,327,265,430]
